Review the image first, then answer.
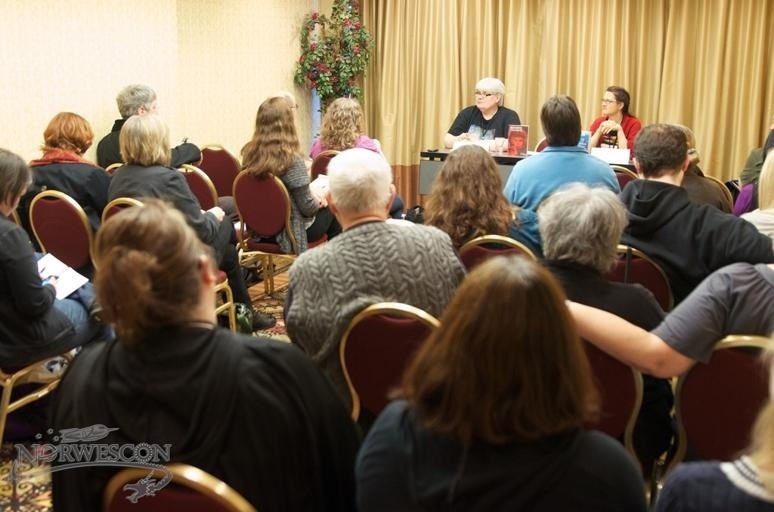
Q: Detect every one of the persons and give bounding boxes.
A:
[0,75,773,510]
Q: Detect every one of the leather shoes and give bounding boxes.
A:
[252,310,276,331]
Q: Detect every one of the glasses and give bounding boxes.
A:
[601,99,616,103]
[475,92,493,97]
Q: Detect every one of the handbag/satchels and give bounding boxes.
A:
[219,303,253,333]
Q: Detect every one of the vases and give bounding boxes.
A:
[320,94,357,124]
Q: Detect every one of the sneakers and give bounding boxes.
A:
[89,296,104,317]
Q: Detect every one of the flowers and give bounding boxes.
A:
[293,0,376,113]
